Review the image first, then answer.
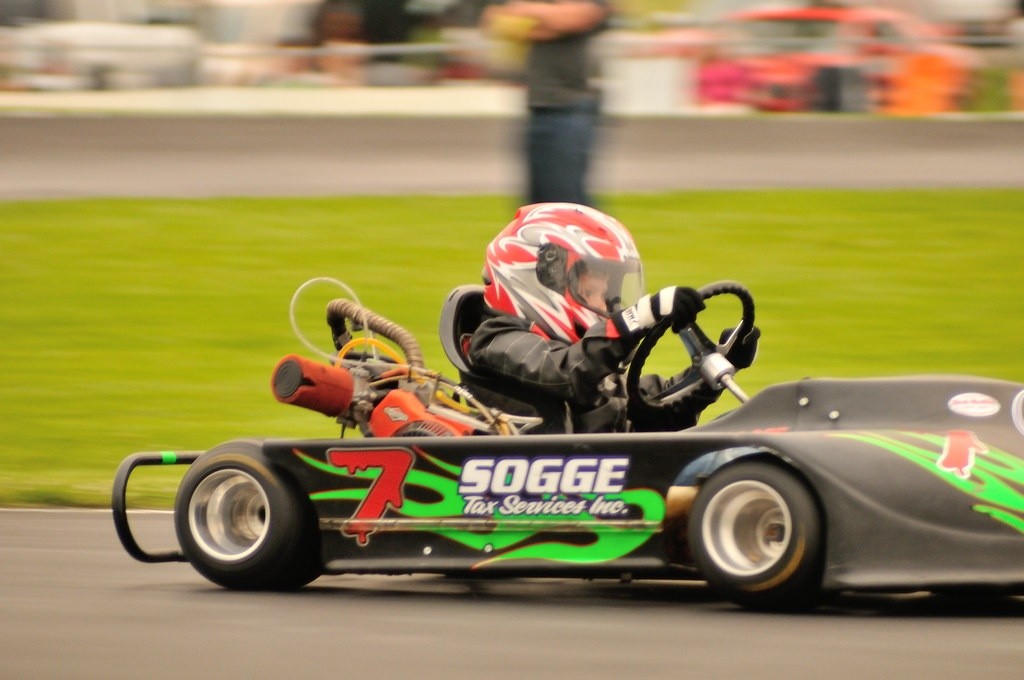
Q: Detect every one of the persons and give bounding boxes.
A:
[462,204,760,434]
[479,0,610,206]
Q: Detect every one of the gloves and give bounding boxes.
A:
[717,326,762,372]
[608,285,706,349]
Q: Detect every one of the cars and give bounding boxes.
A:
[1,1,542,114]
[692,1,1024,120]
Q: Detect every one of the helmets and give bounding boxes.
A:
[481,200,644,349]
